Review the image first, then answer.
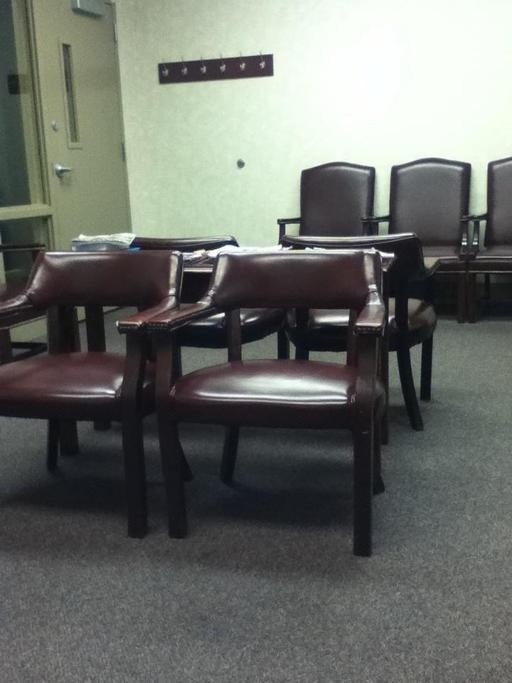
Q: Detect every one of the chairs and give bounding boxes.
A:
[360,159,474,323]
[148,247,388,555]
[1,249,184,538]
[462,157,512,326]
[130,234,281,381]
[277,160,376,244]
[281,233,438,431]
